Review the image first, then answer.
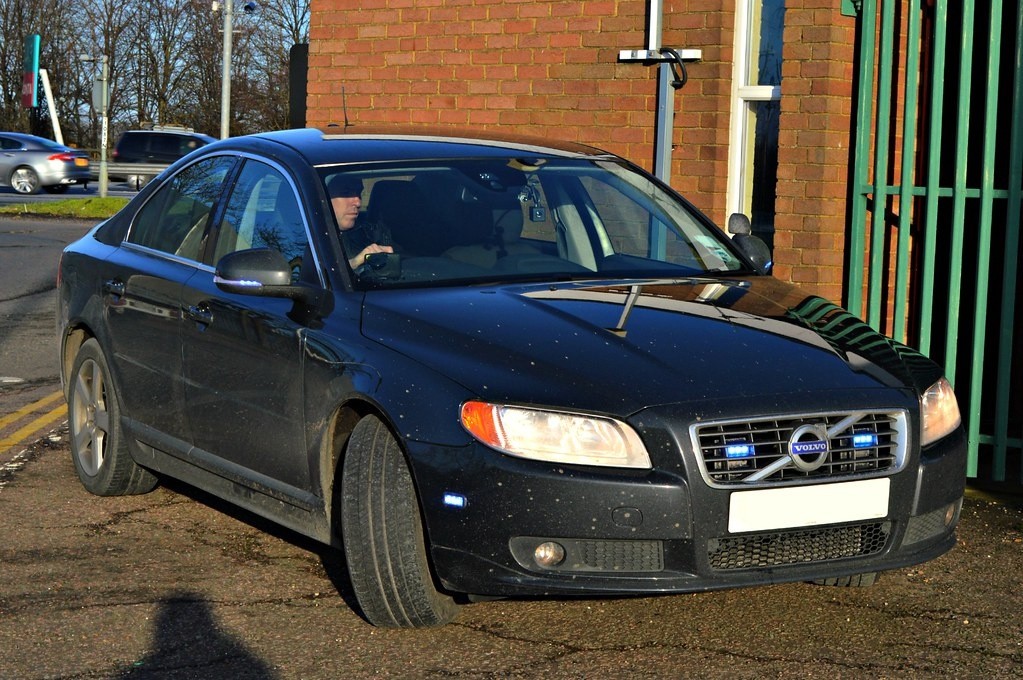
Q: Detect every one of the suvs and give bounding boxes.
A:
[108,129,221,191]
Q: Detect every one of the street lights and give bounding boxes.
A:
[78,54,109,199]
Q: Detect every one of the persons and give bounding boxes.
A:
[76,136,88,191]
[300,175,394,290]
[183,140,202,156]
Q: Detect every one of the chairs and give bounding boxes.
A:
[449,194,541,266]
[369,179,413,236]
[259,182,308,253]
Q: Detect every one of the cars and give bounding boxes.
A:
[0,132,93,195]
[57,126,969,631]
